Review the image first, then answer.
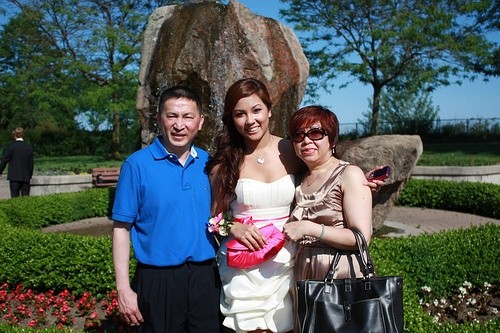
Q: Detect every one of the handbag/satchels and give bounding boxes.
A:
[296,227,404,333]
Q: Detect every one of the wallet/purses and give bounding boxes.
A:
[226,224,286,269]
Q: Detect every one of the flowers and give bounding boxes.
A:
[208,211,232,237]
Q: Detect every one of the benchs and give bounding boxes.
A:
[92,167,121,220]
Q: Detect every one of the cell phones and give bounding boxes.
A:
[368,166,391,183]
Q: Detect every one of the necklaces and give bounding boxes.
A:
[257,156,264,164]
[319,224,325,239]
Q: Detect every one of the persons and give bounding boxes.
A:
[204,78,385,333]
[112,86,224,333]
[282,106,372,333]
[0,127,34,199]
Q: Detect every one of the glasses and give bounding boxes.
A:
[291,127,326,143]
